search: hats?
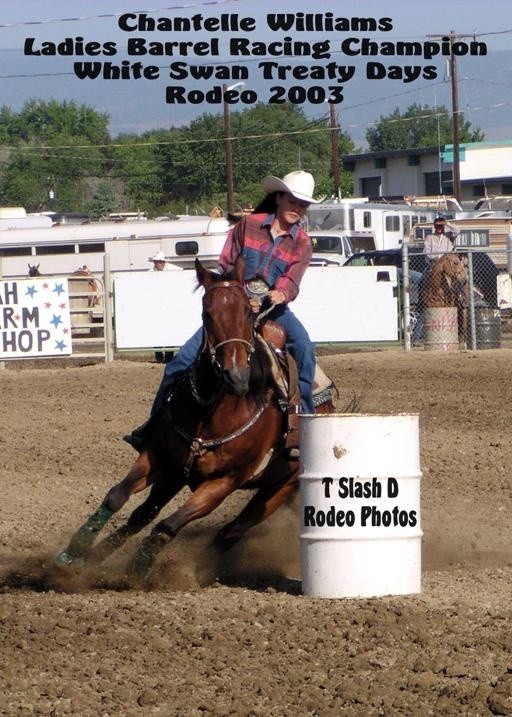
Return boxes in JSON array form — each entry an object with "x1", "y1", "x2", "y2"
[{"x1": 262, "y1": 170, "x2": 328, "y2": 204}]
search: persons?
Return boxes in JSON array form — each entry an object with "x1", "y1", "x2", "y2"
[
  {"x1": 146, "y1": 250, "x2": 175, "y2": 363},
  {"x1": 124, "y1": 169, "x2": 330, "y2": 460},
  {"x1": 457, "y1": 252, "x2": 485, "y2": 350},
  {"x1": 414, "y1": 217, "x2": 461, "y2": 313}
]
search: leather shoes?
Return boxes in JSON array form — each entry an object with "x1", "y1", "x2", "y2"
[{"x1": 122, "y1": 433, "x2": 141, "y2": 445}]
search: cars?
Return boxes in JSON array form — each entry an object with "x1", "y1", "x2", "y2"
[
  {"x1": 306, "y1": 231, "x2": 353, "y2": 266},
  {"x1": 343, "y1": 248, "x2": 499, "y2": 342}
]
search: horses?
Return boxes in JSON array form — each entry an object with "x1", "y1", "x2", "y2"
[
  {"x1": 416, "y1": 248, "x2": 469, "y2": 349},
  {"x1": 55, "y1": 251, "x2": 340, "y2": 582},
  {"x1": 70, "y1": 263, "x2": 100, "y2": 323},
  {"x1": 27, "y1": 262, "x2": 42, "y2": 277}
]
